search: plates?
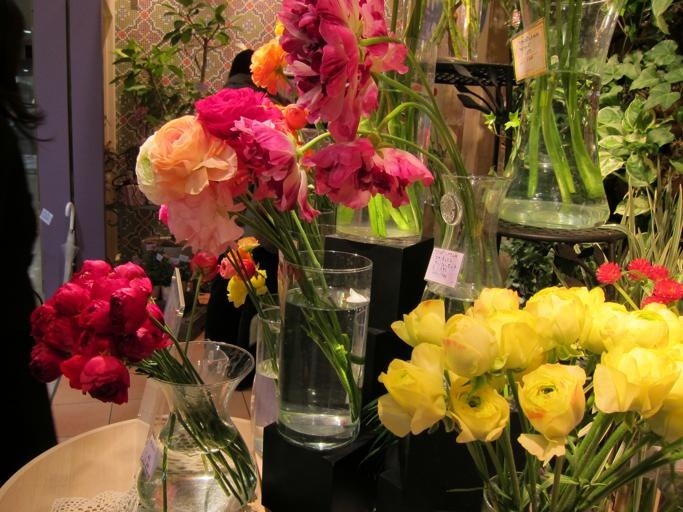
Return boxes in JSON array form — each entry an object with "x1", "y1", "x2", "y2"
[{"x1": 0, "y1": 410, "x2": 265, "y2": 512}]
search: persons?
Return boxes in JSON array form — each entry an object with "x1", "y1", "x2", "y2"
[{"x1": 2, "y1": 1, "x2": 58, "y2": 489}]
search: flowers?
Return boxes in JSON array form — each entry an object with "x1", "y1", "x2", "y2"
[{"x1": 22, "y1": 2, "x2": 680, "y2": 512}]
